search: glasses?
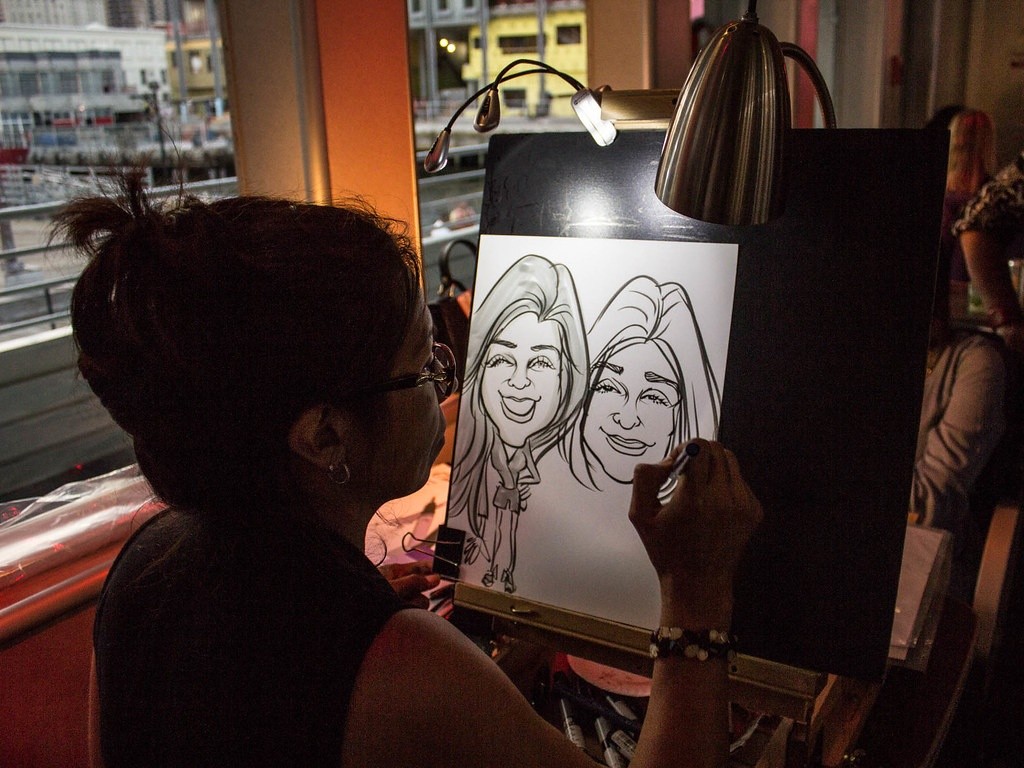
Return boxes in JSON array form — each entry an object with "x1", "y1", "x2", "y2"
[{"x1": 337, "y1": 343, "x2": 457, "y2": 406}]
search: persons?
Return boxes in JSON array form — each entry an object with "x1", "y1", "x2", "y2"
[
  {"x1": 922, "y1": 103, "x2": 1024, "y2": 352},
  {"x1": 908, "y1": 244, "x2": 1005, "y2": 523},
  {"x1": 57, "y1": 195, "x2": 762, "y2": 767}
]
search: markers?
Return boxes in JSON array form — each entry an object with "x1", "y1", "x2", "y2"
[
  {"x1": 600, "y1": 688, "x2": 643, "y2": 728},
  {"x1": 593, "y1": 715, "x2": 627, "y2": 768},
  {"x1": 606, "y1": 728, "x2": 638, "y2": 762},
  {"x1": 559, "y1": 697, "x2": 588, "y2": 753},
  {"x1": 671, "y1": 442, "x2": 700, "y2": 475}
]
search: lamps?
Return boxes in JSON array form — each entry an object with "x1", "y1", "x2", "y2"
[
  {"x1": 424, "y1": 59, "x2": 616, "y2": 172},
  {"x1": 653, "y1": 6, "x2": 833, "y2": 229}
]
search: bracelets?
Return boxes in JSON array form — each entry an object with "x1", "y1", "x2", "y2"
[
  {"x1": 649, "y1": 627, "x2": 735, "y2": 660},
  {"x1": 992, "y1": 317, "x2": 1020, "y2": 332}
]
